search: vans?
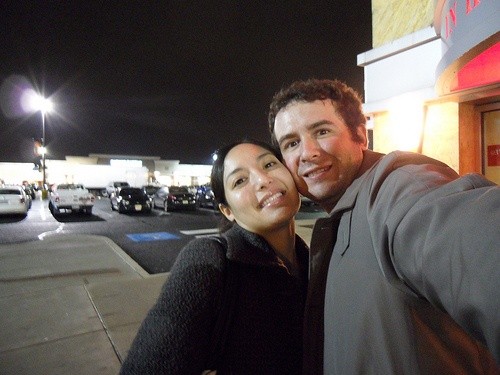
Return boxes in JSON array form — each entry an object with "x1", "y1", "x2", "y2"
[{"x1": 105, "y1": 182, "x2": 129, "y2": 197}]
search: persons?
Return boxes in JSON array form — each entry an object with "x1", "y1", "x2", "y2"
[
  {"x1": 258, "y1": 76, "x2": 500, "y2": 374},
  {"x1": 33, "y1": 138, "x2": 43, "y2": 173},
  {"x1": 116, "y1": 140, "x2": 322, "y2": 374}
]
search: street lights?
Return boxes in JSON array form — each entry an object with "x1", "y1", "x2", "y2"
[{"x1": 30, "y1": 98, "x2": 53, "y2": 191}]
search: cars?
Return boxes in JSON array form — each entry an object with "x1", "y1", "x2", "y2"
[
  {"x1": 142, "y1": 186, "x2": 161, "y2": 203},
  {"x1": 112, "y1": 187, "x2": 151, "y2": 215},
  {"x1": 189, "y1": 185, "x2": 220, "y2": 212},
  {"x1": 150, "y1": 186, "x2": 196, "y2": 214},
  {"x1": 0, "y1": 180, "x2": 35, "y2": 219}
]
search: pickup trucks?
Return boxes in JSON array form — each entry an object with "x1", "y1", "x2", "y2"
[{"x1": 48, "y1": 182, "x2": 93, "y2": 219}]
